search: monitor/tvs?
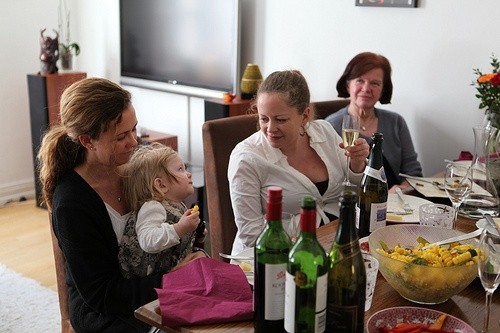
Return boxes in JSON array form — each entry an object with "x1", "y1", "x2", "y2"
[{"x1": 119, "y1": 0, "x2": 243, "y2": 98}]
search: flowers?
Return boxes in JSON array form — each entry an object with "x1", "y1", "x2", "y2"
[{"x1": 468, "y1": 52, "x2": 500, "y2": 152}]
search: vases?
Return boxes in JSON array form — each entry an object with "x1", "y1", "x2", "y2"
[{"x1": 484, "y1": 125, "x2": 500, "y2": 166}]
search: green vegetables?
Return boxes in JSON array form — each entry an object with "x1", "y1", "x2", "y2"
[{"x1": 379, "y1": 236, "x2": 477, "y2": 289}]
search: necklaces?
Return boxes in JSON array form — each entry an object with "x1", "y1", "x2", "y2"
[
  {"x1": 345, "y1": 111, "x2": 375, "y2": 131},
  {"x1": 111, "y1": 189, "x2": 123, "y2": 201}
]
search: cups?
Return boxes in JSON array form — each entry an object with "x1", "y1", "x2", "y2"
[
  {"x1": 362, "y1": 254, "x2": 379, "y2": 312},
  {"x1": 419, "y1": 204, "x2": 455, "y2": 229}
]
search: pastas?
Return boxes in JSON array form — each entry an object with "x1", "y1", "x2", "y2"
[{"x1": 374, "y1": 243, "x2": 489, "y2": 291}]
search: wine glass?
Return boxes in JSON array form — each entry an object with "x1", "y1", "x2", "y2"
[
  {"x1": 338, "y1": 115, "x2": 360, "y2": 187},
  {"x1": 478, "y1": 225, "x2": 500, "y2": 333},
  {"x1": 445, "y1": 164, "x2": 473, "y2": 230}
]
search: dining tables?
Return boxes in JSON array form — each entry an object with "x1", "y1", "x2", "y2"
[{"x1": 134, "y1": 168, "x2": 500, "y2": 333}]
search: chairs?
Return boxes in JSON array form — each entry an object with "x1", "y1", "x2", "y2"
[{"x1": 49, "y1": 99, "x2": 352, "y2": 333}]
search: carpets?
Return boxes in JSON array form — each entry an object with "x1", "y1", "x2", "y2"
[{"x1": 0, "y1": 261, "x2": 62, "y2": 333}]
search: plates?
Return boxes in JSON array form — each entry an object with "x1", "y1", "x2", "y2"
[
  {"x1": 450, "y1": 161, "x2": 487, "y2": 180},
  {"x1": 231, "y1": 248, "x2": 254, "y2": 286},
  {"x1": 476, "y1": 218, "x2": 500, "y2": 237},
  {"x1": 406, "y1": 178, "x2": 493, "y2": 197},
  {"x1": 366, "y1": 307, "x2": 476, "y2": 333},
  {"x1": 358, "y1": 235, "x2": 369, "y2": 254},
  {"x1": 386, "y1": 194, "x2": 441, "y2": 222}
]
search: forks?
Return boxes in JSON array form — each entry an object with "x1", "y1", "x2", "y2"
[{"x1": 395, "y1": 186, "x2": 414, "y2": 210}]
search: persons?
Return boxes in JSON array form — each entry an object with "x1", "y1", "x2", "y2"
[
  {"x1": 36, "y1": 77, "x2": 211, "y2": 333},
  {"x1": 116, "y1": 142, "x2": 208, "y2": 276},
  {"x1": 230, "y1": 70, "x2": 370, "y2": 294},
  {"x1": 325, "y1": 52, "x2": 423, "y2": 196}
]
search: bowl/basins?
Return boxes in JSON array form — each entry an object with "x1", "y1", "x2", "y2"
[{"x1": 369, "y1": 224, "x2": 490, "y2": 304}]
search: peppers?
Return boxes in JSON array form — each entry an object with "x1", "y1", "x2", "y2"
[{"x1": 379, "y1": 323, "x2": 455, "y2": 333}]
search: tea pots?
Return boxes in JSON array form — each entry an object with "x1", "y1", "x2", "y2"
[{"x1": 223, "y1": 93, "x2": 236, "y2": 102}]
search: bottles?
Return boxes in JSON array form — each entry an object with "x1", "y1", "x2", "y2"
[
  {"x1": 454, "y1": 125, "x2": 500, "y2": 219},
  {"x1": 253, "y1": 186, "x2": 292, "y2": 333},
  {"x1": 327, "y1": 191, "x2": 366, "y2": 333},
  {"x1": 284, "y1": 196, "x2": 328, "y2": 333},
  {"x1": 358, "y1": 133, "x2": 388, "y2": 239}
]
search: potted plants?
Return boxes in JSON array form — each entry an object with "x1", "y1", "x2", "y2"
[{"x1": 57, "y1": 42, "x2": 81, "y2": 71}]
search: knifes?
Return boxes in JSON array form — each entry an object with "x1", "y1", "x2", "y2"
[
  {"x1": 387, "y1": 211, "x2": 413, "y2": 215},
  {"x1": 484, "y1": 213, "x2": 500, "y2": 235}
]
